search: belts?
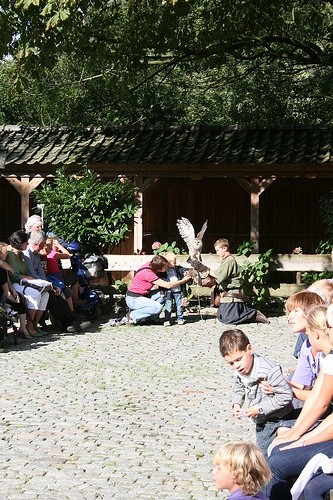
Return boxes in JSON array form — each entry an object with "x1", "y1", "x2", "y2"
[{"x1": 221, "y1": 292, "x2": 245, "y2": 300}]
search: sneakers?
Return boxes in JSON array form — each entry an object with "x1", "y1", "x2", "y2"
[{"x1": 255, "y1": 310, "x2": 269, "y2": 323}]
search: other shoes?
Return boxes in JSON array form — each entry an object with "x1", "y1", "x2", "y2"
[
  {"x1": 164, "y1": 321, "x2": 170, "y2": 326},
  {"x1": 178, "y1": 319, "x2": 184, "y2": 325},
  {"x1": 66, "y1": 321, "x2": 91, "y2": 332},
  {"x1": 27, "y1": 327, "x2": 43, "y2": 337},
  {"x1": 19, "y1": 327, "x2": 33, "y2": 339},
  {"x1": 34, "y1": 327, "x2": 48, "y2": 336},
  {"x1": 126, "y1": 312, "x2": 135, "y2": 326}
]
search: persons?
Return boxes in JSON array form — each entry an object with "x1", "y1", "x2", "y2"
[
  {"x1": 212, "y1": 441, "x2": 272, "y2": 500},
  {"x1": 156, "y1": 253, "x2": 190, "y2": 327},
  {"x1": 126, "y1": 255, "x2": 192, "y2": 328},
  {"x1": 265, "y1": 278, "x2": 333, "y2": 500},
  {"x1": 22, "y1": 234, "x2": 91, "y2": 332},
  {"x1": 219, "y1": 329, "x2": 297, "y2": 461},
  {"x1": 4, "y1": 230, "x2": 49, "y2": 337},
  {"x1": 0, "y1": 242, "x2": 32, "y2": 338},
  {"x1": 194, "y1": 238, "x2": 270, "y2": 326},
  {"x1": 24, "y1": 215, "x2": 87, "y2": 322}
]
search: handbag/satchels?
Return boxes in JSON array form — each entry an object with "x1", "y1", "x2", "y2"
[{"x1": 20, "y1": 278, "x2": 53, "y2": 293}]
